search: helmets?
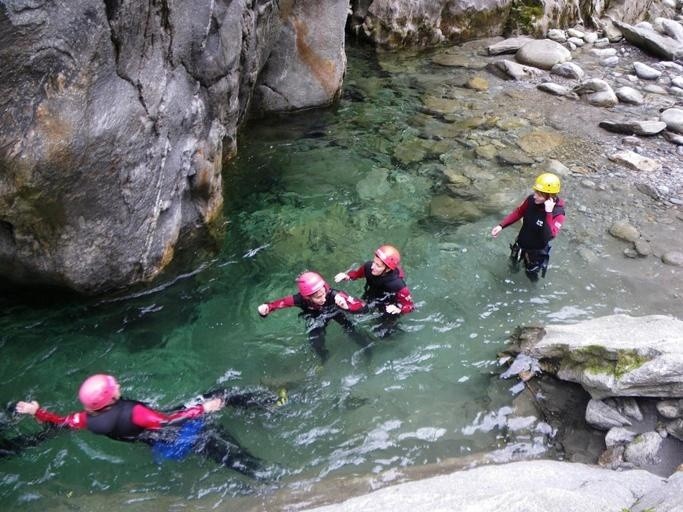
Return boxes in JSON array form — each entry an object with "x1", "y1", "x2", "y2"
[
  {"x1": 532, "y1": 173, "x2": 560, "y2": 193},
  {"x1": 77, "y1": 373, "x2": 117, "y2": 411},
  {"x1": 375, "y1": 245, "x2": 400, "y2": 271},
  {"x1": 298, "y1": 272, "x2": 325, "y2": 297}
]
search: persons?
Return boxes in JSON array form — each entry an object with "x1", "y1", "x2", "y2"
[
  {"x1": 334, "y1": 245, "x2": 417, "y2": 341},
  {"x1": 491, "y1": 174, "x2": 565, "y2": 286},
  {"x1": 259, "y1": 272, "x2": 377, "y2": 367},
  {"x1": 17, "y1": 373, "x2": 292, "y2": 485}
]
